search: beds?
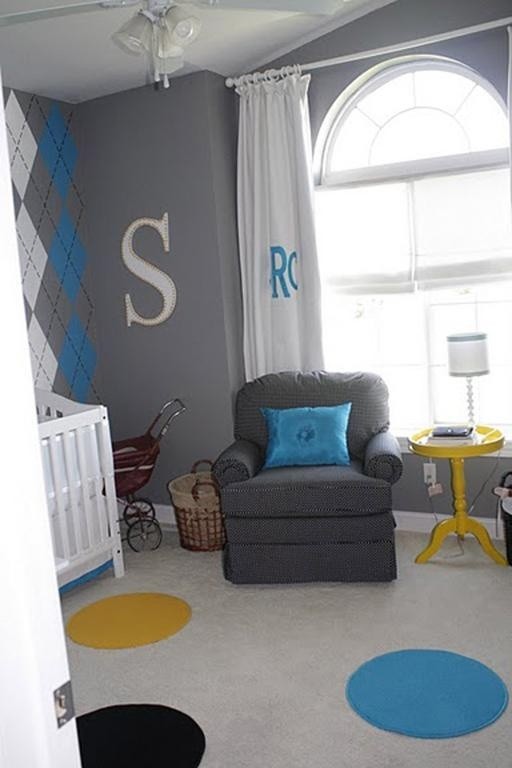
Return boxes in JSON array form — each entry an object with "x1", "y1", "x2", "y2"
[{"x1": 34, "y1": 388, "x2": 125, "y2": 595}]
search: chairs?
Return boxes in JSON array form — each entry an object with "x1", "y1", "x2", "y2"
[{"x1": 213, "y1": 370, "x2": 403, "y2": 585}]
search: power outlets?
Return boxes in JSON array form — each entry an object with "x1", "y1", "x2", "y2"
[{"x1": 424, "y1": 463, "x2": 436, "y2": 483}]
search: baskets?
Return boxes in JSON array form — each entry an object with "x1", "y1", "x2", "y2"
[{"x1": 168, "y1": 471, "x2": 225, "y2": 551}]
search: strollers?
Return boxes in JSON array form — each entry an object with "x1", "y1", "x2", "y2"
[{"x1": 101, "y1": 397, "x2": 187, "y2": 552}]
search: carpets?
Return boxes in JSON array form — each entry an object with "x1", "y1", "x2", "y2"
[
  {"x1": 76, "y1": 704, "x2": 205, "y2": 768},
  {"x1": 346, "y1": 650, "x2": 508, "y2": 740},
  {"x1": 66, "y1": 593, "x2": 192, "y2": 650}
]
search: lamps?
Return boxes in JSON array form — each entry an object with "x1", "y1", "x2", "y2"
[
  {"x1": 450, "y1": 334, "x2": 490, "y2": 427},
  {"x1": 114, "y1": 5, "x2": 203, "y2": 88}
]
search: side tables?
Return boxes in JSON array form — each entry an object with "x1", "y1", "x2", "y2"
[{"x1": 408, "y1": 425, "x2": 506, "y2": 563}]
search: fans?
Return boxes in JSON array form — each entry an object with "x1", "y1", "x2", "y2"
[{"x1": 0, "y1": 1, "x2": 345, "y2": 26}]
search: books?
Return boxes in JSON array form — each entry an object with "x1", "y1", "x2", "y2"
[
  {"x1": 432, "y1": 424, "x2": 473, "y2": 437},
  {"x1": 428, "y1": 426, "x2": 477, "y2": 440}
]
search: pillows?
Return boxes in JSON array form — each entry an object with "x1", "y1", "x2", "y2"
[{"x1": 259, "y1": 402, "x2": 354, "y2": 471}]
